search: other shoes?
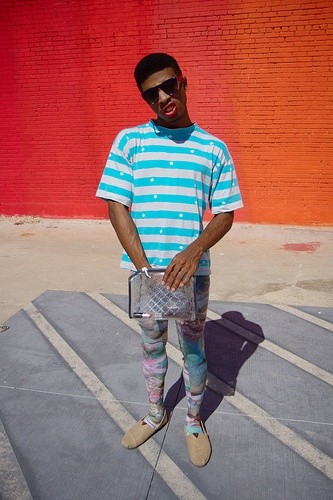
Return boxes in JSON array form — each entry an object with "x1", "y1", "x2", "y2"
[
  {"x1": 121, "y1": 409, "x2": 168, "y2": 449},
  {"x1": 184, "y1": 418, "x2": 212, "y2": 467}
]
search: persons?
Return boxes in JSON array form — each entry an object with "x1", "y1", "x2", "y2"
[{"x1": 94, "y1": 51, "x2": 244, "y2": 469}]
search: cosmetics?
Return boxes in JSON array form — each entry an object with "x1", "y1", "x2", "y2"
[{"x1": 161, "y1": 310, "x2": 189, "y2": 319}]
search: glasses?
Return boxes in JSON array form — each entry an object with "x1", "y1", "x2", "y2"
[{"x1": 141, "y1": 72, "x2": 182, "y2": 105}]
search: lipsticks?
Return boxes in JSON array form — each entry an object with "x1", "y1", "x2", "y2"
[{"x1": 132, "y1": 312, "x2": 150, "y2": 318}]
